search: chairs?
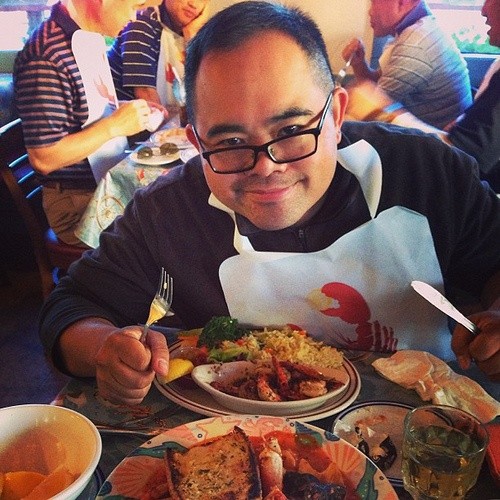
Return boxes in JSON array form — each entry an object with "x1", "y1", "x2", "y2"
[{"x1": 0, "y1": 117, "x2": 94, "y2": 303}]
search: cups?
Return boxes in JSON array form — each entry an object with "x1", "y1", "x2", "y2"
[{"x1": 399, "y1": 404, "x2": 490, "y2": 500}]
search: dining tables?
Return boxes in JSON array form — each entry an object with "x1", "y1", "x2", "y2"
[
  {"x1": 110, "y1": 110, "x2": 201, "y2": 193},
  {"x1": 0, "y1": 309, "x2": 500, "y2": 500}
]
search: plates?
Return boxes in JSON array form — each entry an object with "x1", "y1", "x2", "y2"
[
  {"x1": 130, "y1": 145, "x2": 181, "y2": 165},
  {"x1": 154, "y1": 330, "x2": 362, "y2": 421},
  {"x1": 327, "y1": 400, "x2": 469, "y2": 490},
  {"x1": 93, "y1": 415, "x2": 399, "y2": 500},
  {"x1": 181, "y1": 149, "x2": 201, "y2": 165},
  {"x1": 150, "y1": 128, "x2": 196, "y2": 149},
  {"x1": 192, "y1": 363, "x2": 350, "y2": 415}
]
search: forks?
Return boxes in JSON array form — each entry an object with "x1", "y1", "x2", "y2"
[
  {"x1": 139, "y1": 267, "x2": 175, "y2": 342},
  {"x1": 334, "y1": 39, "x2": 362, "y2": 88}
]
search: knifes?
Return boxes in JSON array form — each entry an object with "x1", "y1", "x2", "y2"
[{"x1": 411, "y1": 280, "x2": 482, "y2": 337}]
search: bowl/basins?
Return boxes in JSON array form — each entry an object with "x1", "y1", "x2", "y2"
[{"x1": 0, "y1": 399, "x2": 102, "y2": 500}]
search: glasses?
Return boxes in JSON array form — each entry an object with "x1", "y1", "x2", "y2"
[{"x1": 191, "y1": 92, "x2": 333, "y2": 175}]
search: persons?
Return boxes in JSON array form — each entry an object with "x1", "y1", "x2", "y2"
[
  {"x1": 341, "y1": 0, "x2": 474, "y2": 130},
  {"x1": 39, "y1": 0, "x2": 500, "y2": 405},
  {"x1": 108, "y1": 0, "x2": 206, "y2": 147},
  {"x1": 12, "y1": 0, "x2": 171, "y2": 246},
  {"x1": 342, "y1": 1, "x2": 500, "y2": 196}
]
